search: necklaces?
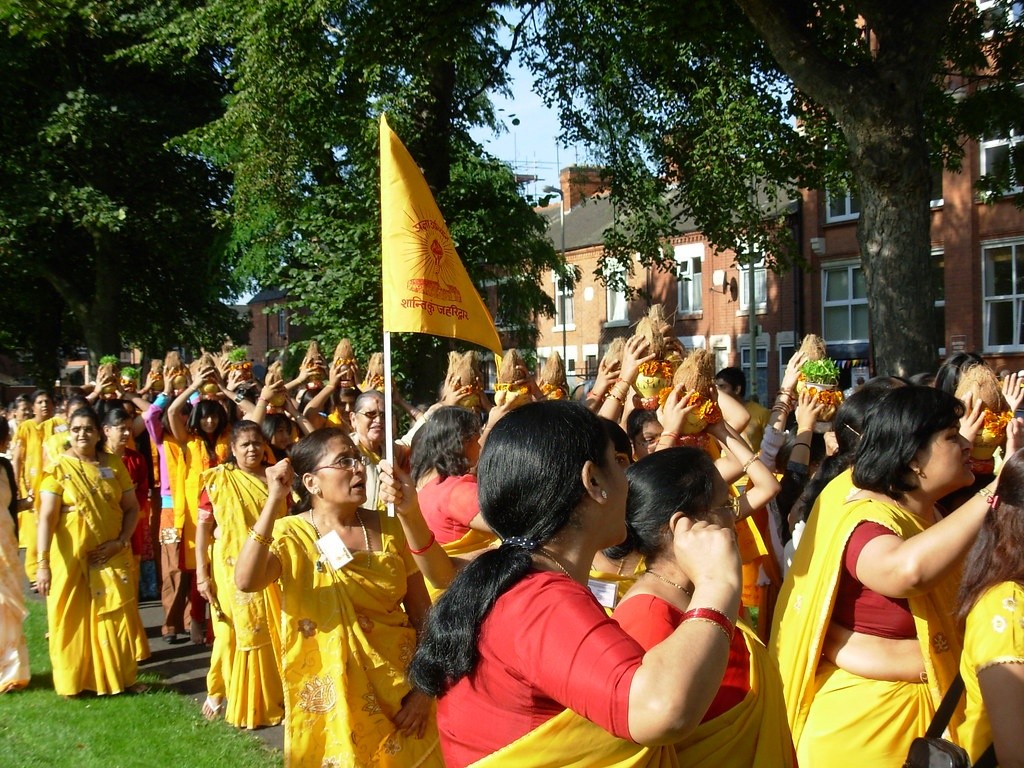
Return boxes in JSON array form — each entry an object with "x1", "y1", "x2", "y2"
[
  {"x1": 646, "y1": 570, "x2": 692, "y2": 598},
  {"x1": 79, "y1": 455, "x2": 101, "y2": 494},
  {"x1": 309, "y1": 508, "x2": 370, "y2": 568},
  {"x1": 538, "y1": 549, "x2": 573, "y2": 580}
]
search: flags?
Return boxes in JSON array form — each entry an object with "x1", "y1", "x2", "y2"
[{"x1": 380, "y1": 115, "x2": 504, "y2": 356}]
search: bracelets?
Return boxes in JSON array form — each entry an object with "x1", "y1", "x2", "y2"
[
  {"x1": 772, "y1": 406, "x2": 788, "y2": 415},
  {"x1": 775, "y1": 401, "x2": 790, "y2": 411},
  {"x1": 979, "y1": 488, "x2": 994, "y2": 499},
  {"x1": 196, "y1": 577, "x2": 210, "y2": 585},
  {"x1": 743, "y1": 456, "x2": 758, "y2": 472},
  {"x1": 777, "y1": 388, "x2": 793, "y2": 398},
  {"x1": 189, "y1": 385, "x2": 198, "y2": 391},
  {"x1": 295, "y1": 413, "x2": 303, "y2": 420},
  {"x1": 661, "y1": 431, "x2": 680, "y2": 440},
  {"x1": 406, "y1": 530, "x2": 435, "y2": 554},
  {"x1": 37, "y1": 552, "x2": 50, "y2": 568},
  {"x1": 793, "y1": 443, "x2": 811, "y2": 450},
  {"x1": 411, "y1": 408, "x2": 420, "y2": 417},
  {"x1": 234, "y1": 395, "x2": 245, "y2": 404},
  {"x1": 604, "y1": 393, "x2": 624, "y2": 406},
  {"x1": 614, "y1": 386, "x2": 626, "y2": 400},
  {"x1": 679, "y1": 608, "x2": 735, "y2": 642},
  {"x1": 257, "y1": 398, "x2": 269, "y2": 403},
  {"x1": 248, "y1": 528, "x2": 274, "y2": 546}
]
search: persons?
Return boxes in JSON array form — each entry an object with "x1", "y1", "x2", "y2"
[{"x1": 0, "y1": 314, "x2": 1024, "y2": 768}]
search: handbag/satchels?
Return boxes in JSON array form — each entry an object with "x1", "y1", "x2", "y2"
[{"x1": 903, "y1": 738, "x2": 972, "y2": 768}]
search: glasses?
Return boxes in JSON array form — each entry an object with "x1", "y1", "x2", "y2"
[
  {"x1": 69, "y1": 426, "x2": 98, "y2": 433},
  {"x1": 354, "y1": 409, "x2": 384, "y2": 418},
  {"x1": 309, "y1": 455, "x2": 371, "y2": 473},
  {"x1": 698, "y1": 498, "x2": 742, "y2": 518},
  {"x1": 634, "y1": 438, "x2": 662, "y2": 450}
]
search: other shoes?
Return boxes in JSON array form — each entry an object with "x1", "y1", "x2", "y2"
[
  {"x1": 30, "y1": 579, "x2": 40, "y2": 592},
  {"x1": 6, "y1": 685, "x2": 19, "y2": 694},
  {"x1": 163, "y1": 634, "x2": 176, "y2": 644}
]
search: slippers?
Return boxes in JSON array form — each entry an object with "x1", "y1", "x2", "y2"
[{"x1": 202, "y1": 693, "x2": 223, "y2": 724}]
